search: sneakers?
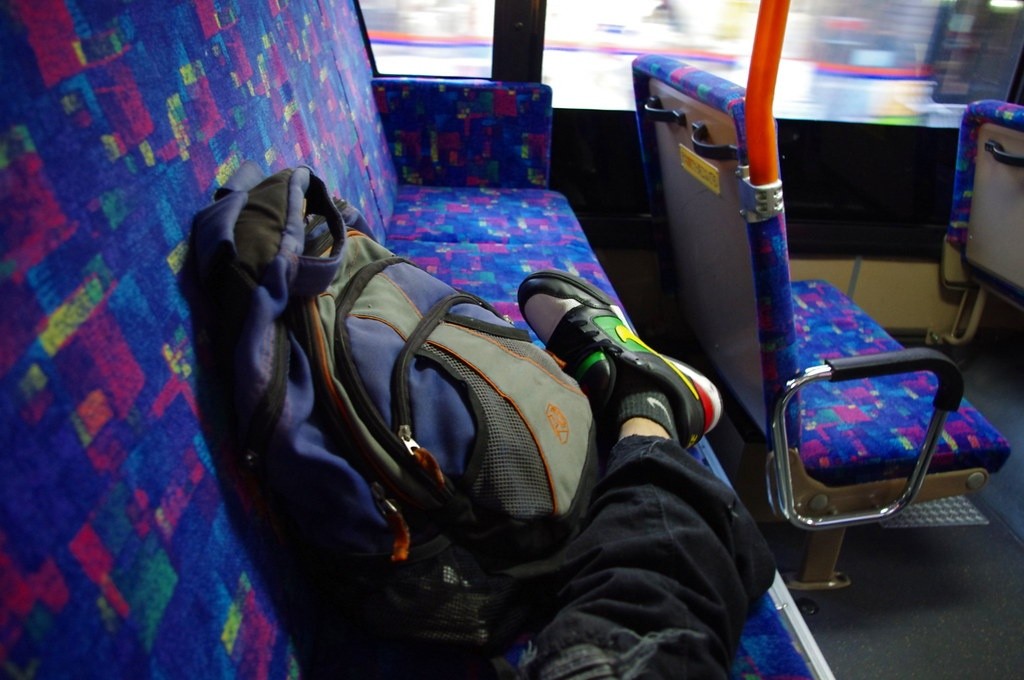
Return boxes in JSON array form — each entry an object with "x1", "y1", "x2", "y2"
[{"x1": 518, "y1": 267, "x2": 724, "y2": 451}]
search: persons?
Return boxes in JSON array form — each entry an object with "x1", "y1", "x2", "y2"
[{"x1": 503, "y1": 269, "x2": 779, "y2": 679}]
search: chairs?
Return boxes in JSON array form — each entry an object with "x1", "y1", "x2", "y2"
[
  {"x1": 626, "y1": 53, "x2": 1016, "y2": 597},
  {"x1": 926, "y1": 90, "x2": 1024, "y2": 340}
]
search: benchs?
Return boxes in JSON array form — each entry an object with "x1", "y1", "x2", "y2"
[{"x1": 0, "y1": 0, "x2": 838, "y2": 680}]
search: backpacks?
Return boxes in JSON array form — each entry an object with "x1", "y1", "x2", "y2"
[{"x1": 182, "y1": 165, "x2": 605, "y2": 653}]
